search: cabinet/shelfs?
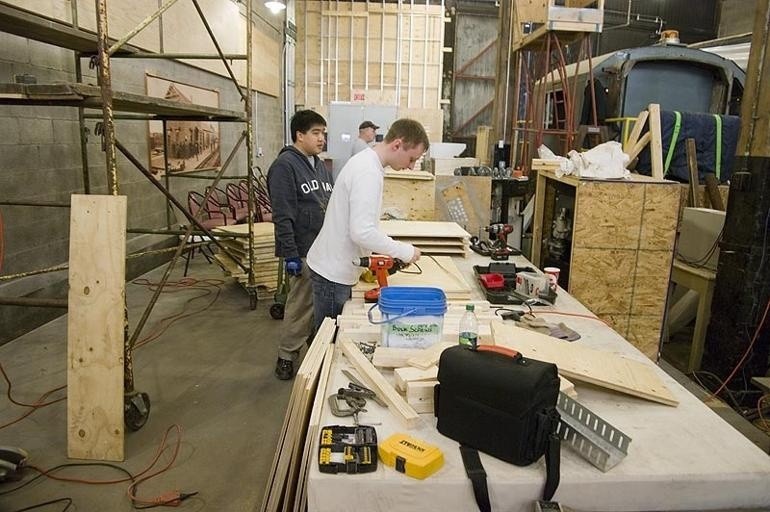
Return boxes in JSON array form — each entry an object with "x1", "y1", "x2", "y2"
[{"x1": 531, "y1": 170, "x2": 682, "y2": 363}]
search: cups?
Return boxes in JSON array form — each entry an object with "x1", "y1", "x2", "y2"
[{"x1": 544, "y1": 267, "x2": 560, "y2": 293}]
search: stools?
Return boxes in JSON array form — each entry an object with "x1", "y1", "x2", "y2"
[{"x1": 672, "y1": 259, "x2": 715, "y2": 379}]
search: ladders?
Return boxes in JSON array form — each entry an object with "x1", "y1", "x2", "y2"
[{"x1": 550, "y1": 30, "x2": 568, "y2": 157}]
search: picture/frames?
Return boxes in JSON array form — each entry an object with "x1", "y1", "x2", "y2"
[{"x1": 145, "y1": 72, "x2": 222, "y2": 178}]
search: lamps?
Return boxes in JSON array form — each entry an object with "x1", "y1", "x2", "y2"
[{"x1": 265, "y1": 1, "x2": 286, "y2": 10}]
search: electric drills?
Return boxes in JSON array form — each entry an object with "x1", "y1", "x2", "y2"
[
  {"x1": 352, "y1": 255, "x2": 395, "y2": 304},
  {"x1": 481, "y1": 224, "x2": 514, "y2": 254}
]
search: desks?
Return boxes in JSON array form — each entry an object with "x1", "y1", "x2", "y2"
[{"x1": 305, "y1": 247, "x2": 769, "y2": 512}]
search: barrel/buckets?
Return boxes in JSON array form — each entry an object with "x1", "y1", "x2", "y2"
[{"x1": 366, "y1": 285, "x2": 449, "y2": 350}]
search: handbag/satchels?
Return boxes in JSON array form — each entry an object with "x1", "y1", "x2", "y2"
[
  {"x1": 636, "y1": 108, "x2": 740, "y2": 184},
  {"x1": 433, "y1": 344, "x2": 561, "y2": 467}
]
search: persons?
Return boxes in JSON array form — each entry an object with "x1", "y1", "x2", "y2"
[
  {"x1": 351, "y1": 120, "x2": 380, "y2": 154},
  {"x1": 306, "y1": 118, "x2": 431, "y2": 331},
  {"x1": 266, "y1": 108, "x2": 335, "y2": 382}
]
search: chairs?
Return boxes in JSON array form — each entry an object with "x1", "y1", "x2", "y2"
[{"x1": 187, "y1": 175, "x2": 273, "y2": 264}]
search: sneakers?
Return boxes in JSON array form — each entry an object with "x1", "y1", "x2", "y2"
[{"x1": 276, "y1": 357, "x2": 293, "y2": 380}]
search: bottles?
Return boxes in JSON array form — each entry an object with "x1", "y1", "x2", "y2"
[{"x1": 459, "y1": 303, "x2": 479, "y2": 350}]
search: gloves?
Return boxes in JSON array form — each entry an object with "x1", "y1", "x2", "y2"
[{"x1": 285, "y1": 257, "x2": 304, "y2": 276}]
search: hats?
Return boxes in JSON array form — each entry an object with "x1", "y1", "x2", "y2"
[{"x1": 359, "y1": 120, "x2": 380, "y2": 129}]
